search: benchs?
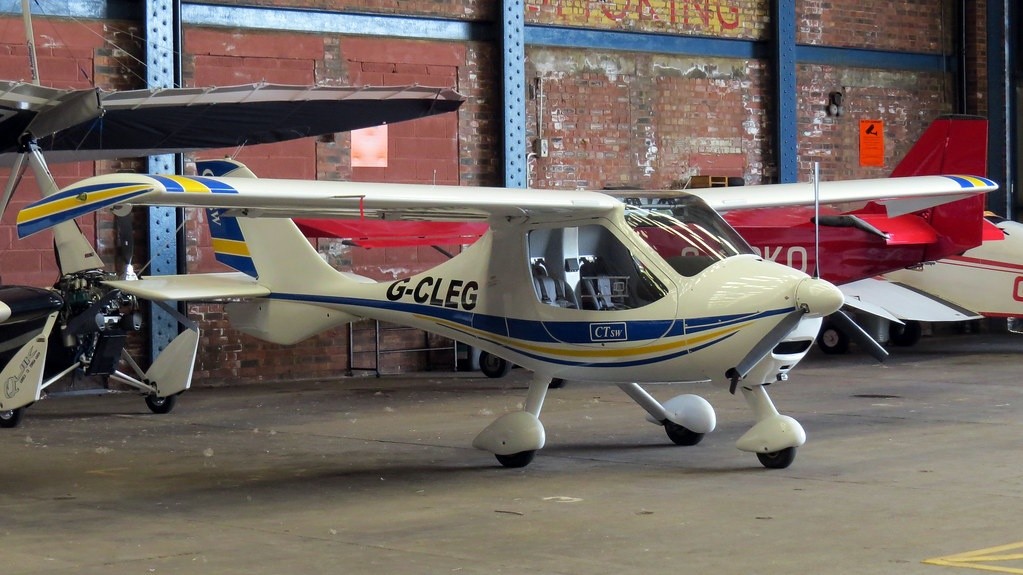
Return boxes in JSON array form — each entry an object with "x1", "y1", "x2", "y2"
[{"x1": 531, "y1": 260, "x2": 580, "y2": 309}]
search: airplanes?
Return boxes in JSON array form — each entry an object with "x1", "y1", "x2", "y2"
[{"x1": 0, "y1": 0, "x2": 1023, "y2": 472}]
